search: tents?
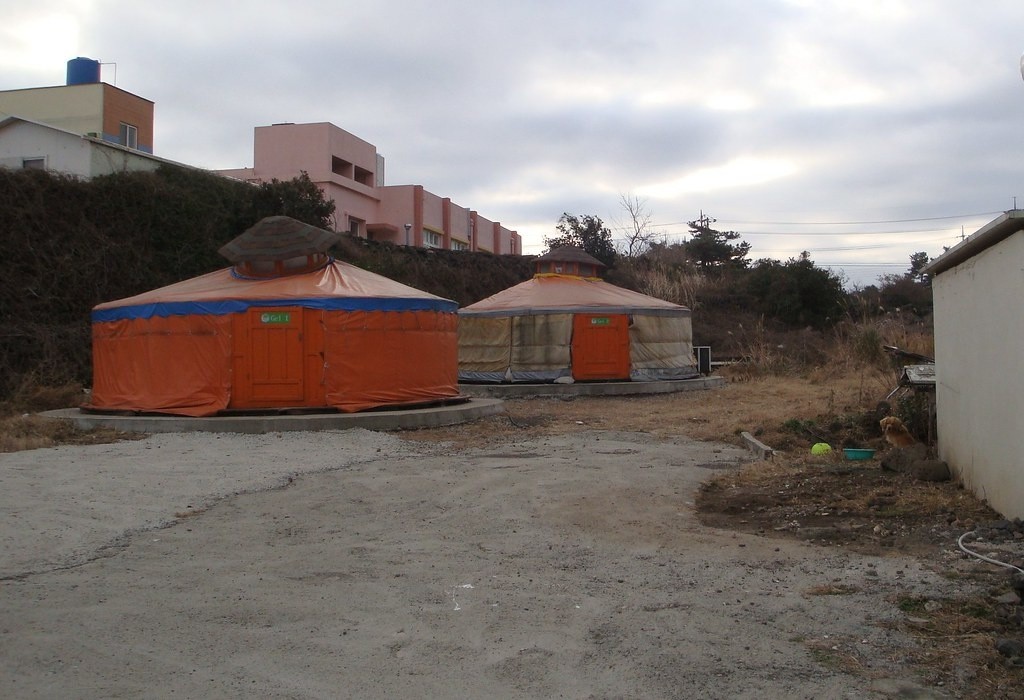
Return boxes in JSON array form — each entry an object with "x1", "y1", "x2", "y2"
[
  {"x1": 90, "y1": 216, "x2": 462, "y2": 417},
  {"x1": 461, "y1": 245, "x2": 697, "y2": 382}
]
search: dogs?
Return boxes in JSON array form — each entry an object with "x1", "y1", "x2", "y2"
[{"x1": 880, "y1": 416, "x2": 917, "y2": 452}]
